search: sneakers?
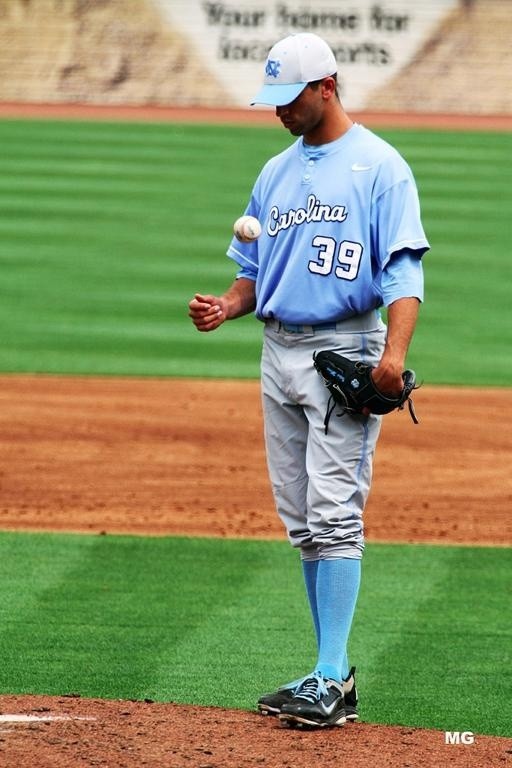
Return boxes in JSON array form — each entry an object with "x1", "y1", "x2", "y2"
[
  {"x1": 258, "y1": 667, "x2": 358, "y2": 721},
  {"x1": 281, "y1": 676, "x2": 345, "y2": 726}
]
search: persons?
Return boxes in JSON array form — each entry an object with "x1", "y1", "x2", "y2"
[{"x1": 188, "y1": 31, "x2": 431, "y2": 730}]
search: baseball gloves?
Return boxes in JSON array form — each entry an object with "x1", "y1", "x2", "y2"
[{"x1": 312, "y1": 350, "x2": 416, "y2": 415}]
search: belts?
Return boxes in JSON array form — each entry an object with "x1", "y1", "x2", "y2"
[{"x1": 278, "y1": 320, "x2": 336, "y2": 333}]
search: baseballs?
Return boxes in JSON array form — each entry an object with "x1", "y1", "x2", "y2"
[{"x1": 233, "y1": 216, "x2": 262, "y2": 243}]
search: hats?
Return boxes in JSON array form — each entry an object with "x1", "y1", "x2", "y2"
[{"x1": 250, "y1": 32, "x2": 336, "y2": 105}]
